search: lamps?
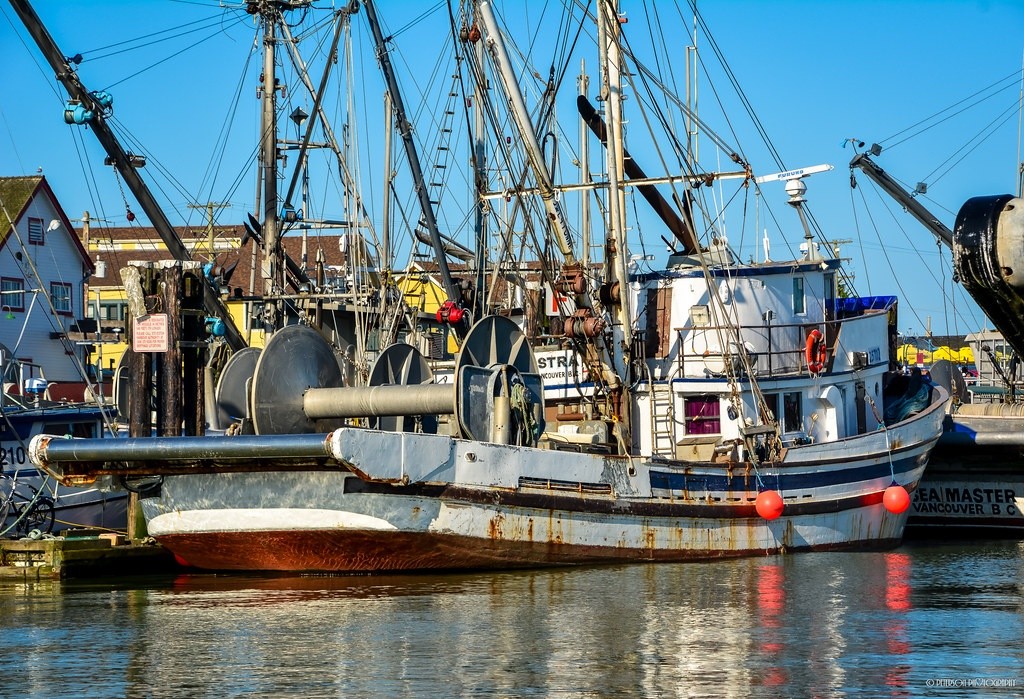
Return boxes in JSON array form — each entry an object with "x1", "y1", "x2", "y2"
[{"x1": 689, "y1": 305, "x2": 712, "y2": 352}]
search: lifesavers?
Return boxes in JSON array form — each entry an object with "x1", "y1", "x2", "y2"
[{"x1": 807, "y1": 330, "x2": 826, "y2": 372}]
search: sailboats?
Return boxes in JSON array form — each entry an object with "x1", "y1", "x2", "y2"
[{"x1": 1, "y1": 0, "x2": 1024, "y2": 583}]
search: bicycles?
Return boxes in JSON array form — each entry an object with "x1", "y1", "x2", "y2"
[{"x1": 0, "y1": 474, "x2": 57, "y2": 538}]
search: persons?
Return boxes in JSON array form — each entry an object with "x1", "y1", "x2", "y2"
[{"x1": 738, "y1": 417, "x2": 757, "y2": 462}]
